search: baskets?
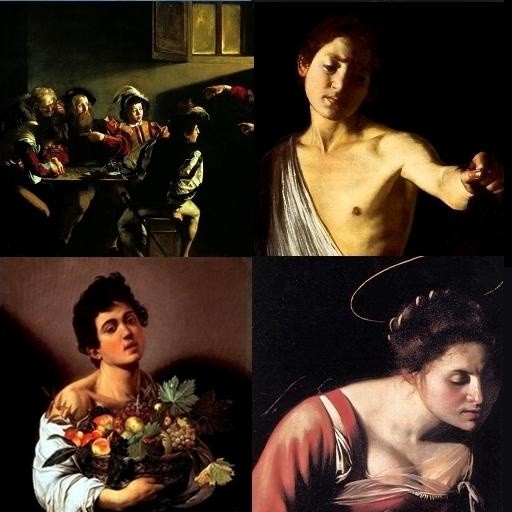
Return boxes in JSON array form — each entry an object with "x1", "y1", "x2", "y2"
[{"x1": 83, "y1": 453, "x2": 192, "y2": 508}]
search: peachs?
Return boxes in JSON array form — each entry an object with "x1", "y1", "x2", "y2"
[
  {"x1": 91, "y1": 437, "x2": 110, "y2": 456},
  {"x1": 93, "y1": 414, "x2": 114, "y2": 430}
]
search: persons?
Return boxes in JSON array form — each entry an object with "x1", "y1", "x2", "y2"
[
  {"x1": 30, "y1": 269, "x2": 217, "y2": 512},
  {"x1": 249, "y1": 289, "x2": 505, "y2": 511},
  {"x1": 267, "y1": 17, "x2": 508, "y2": 256}
]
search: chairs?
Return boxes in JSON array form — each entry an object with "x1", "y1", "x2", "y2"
[{"x1": 119, "y1": 191, "x2": 178, "y2": 256}]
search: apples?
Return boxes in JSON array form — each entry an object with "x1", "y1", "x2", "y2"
[{"x1": 125, "y1": 416, "x2": 145, "y2": 435}]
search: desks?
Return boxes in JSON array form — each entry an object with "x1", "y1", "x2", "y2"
[{"x1": 41, "y1": 164, "x2": 129, "y2": 246}]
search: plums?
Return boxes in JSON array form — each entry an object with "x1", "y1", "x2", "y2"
[{"x1": 65, "y1": 427, "x2": 105, "y2": 447}]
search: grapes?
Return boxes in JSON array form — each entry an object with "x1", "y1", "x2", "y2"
[
  {"x1": 166, "y1": 426, "x2": 197, "y2": 450},
  {"x1": 119, "y1": 400, "x2": 152, "y2": 424}
]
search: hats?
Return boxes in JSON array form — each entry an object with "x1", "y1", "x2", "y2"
[
  {"x1": 168, "y1": 113, "x2": 199, "y2": 141},
  {"x1": 119, "y1": 95, "x2": 150, "y2": 120},
  {"x1": 63, "y1": 87, "x2": 96, "y2": 107}
]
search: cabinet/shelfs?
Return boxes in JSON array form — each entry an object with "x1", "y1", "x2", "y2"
[{"x1": 146, "y1": 1, "x2": 255, "y2": 63}]
